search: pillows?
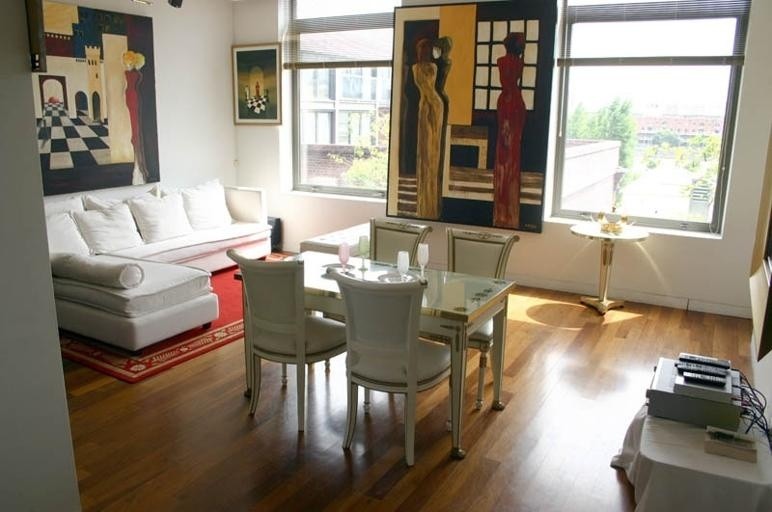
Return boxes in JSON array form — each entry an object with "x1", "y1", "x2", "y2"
[
  {"x1": 72, "y1": 203, "x2": 144, "y2": 257},
  {"x1": 181, "y1": 180, "x2": 234, "y2": 230},
  {"x1": 44, "y1": 213, "x2": 92, "y2": 257},
  {"x1": 128, "y1": 193, "x2": 193, "y2": 243}
]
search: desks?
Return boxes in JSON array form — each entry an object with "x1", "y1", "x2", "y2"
[
  {"x1": 570, "y1": 221, "x2": 651, "y2": 315},
  {"x1": 610, "y1": 385, "x2": 772, "y2": 511}
]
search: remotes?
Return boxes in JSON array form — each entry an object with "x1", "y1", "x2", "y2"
[
  {"x1": 679, "y1": 352, "x2": 731, "y2": 370},
  {"x1": 683, "y1": 371, "x2": 726, "y2": 389},
  {"x1": 674, "y1": 360, "x2": 727, "y2": 378}
]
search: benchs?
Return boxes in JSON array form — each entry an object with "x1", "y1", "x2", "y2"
[{"x1": 300, "y1": 222, "x2": 373, "y2": 258}]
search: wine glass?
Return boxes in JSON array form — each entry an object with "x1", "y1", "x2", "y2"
[
  {"x1": 338, "y1": 240, "x2": 349, "y2": 273},
  {"x1": 418, "y1": 244, "x2": 429, "y2": 280},
  {"x1": 359, "y1": 236, "x2": 369, "y2": 270},
  {"x1": 398, "y1": 251, "x2": 409, "y2": 275}
]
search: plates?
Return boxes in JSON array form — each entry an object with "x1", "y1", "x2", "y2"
[{"x1": 377, "y1": 274, "x2": 415, "y2": 283}]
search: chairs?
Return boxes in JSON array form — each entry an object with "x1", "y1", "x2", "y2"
[
  {"x1": 235, "y1": 251, "x2": 518, "y2": 459},
  {"x1": 225, "y1": 248, "x2": 346, "y2": 436},
  {"x1": 444, "y1": 228, "x2": 520, "y2": 411},
  {"x1": 366, "y1": 218, "x2": 435, "y2": 264},
  {"x1": 331, "y1": 272, "x2": 452, "y2": 465}
]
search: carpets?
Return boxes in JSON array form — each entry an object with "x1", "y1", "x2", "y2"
[{"x1": 61, "y1": 252, "x2": 311, "y2": 385}]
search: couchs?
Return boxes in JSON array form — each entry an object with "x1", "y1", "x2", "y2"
[{"x1": 44, "y1": 183, "x2": 274, "y2": 359}]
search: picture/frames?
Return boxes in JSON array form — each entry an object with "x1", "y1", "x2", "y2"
[
  {"x1": 231, "y1": 41, "x2": 284, "y2": 125},
  {"x1": 385, "y1": 0, "x2": 559, "y2": 234}
]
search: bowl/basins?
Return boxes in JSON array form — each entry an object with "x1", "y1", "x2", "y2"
[{"x1": 606, "y1": 213, "x2": 620, "y2": 224}]
